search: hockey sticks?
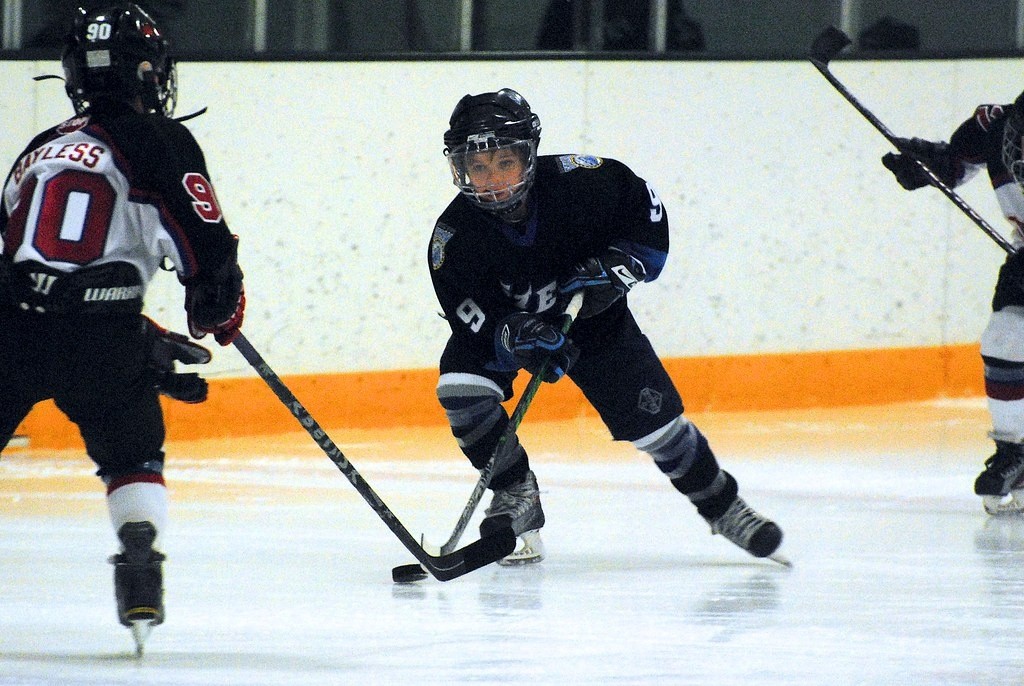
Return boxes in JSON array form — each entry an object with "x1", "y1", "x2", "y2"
[
  {"x1": 420, "y1": 291, "x2": 585, "y2": 579},
  {"x1": 230, "y1": 330, "x2": 518, "y2": 585},
  {"x1": 804, "y1": 22, "x2": 1018, "y2": 255}
]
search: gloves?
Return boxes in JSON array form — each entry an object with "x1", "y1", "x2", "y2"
[
  {"x1": 188, "y1": 291, "x2": 246, "y2": 346},
  {"x1": 883, "y1": 137, "x2": 954, "y2": 191},
  {"x1": 494, "y1": 312, "x2": 579, "y2": 384},
  {"x1": 142, "y1": 318, "x2": 211, "y2": 404},
  {"x1": 556, "y1": 251, "x2": 647, "y2": 318}
]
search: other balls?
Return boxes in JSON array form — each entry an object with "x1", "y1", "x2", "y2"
[{"x1": 391, "y1": 563, "x2": 430, "y2": 584}]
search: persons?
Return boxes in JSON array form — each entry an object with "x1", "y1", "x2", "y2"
[
  {"x1": 427, "y1": 88, "x2": 791, "y2": 568},
  {"x1": 0, "y1": 0, "x2": 246, "y2": 656},
  {"x1": 880, "y1": 92, "x2": 1024, "y2": 518}
]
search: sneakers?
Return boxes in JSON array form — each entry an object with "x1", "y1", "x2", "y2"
[
  {"x1": 480, "y1": 471, "x2": 545, "y2": 564},
  {"x1": 105, "y1": 522, "x2": 168, "y2": 656},
  {"x1": 709, "y1": 495, "x2": 791, "y2": 567},
  {"x1": 973, "y1": 431, "x2": 1024, "y2": 516}
]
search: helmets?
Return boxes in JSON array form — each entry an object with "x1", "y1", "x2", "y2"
[
  {"x1": 59, "y1": 1, "x2": 178, "y2": 117},
  {"x1": 444, "y1": 89, "x2": 542, "y2": 222}
]
